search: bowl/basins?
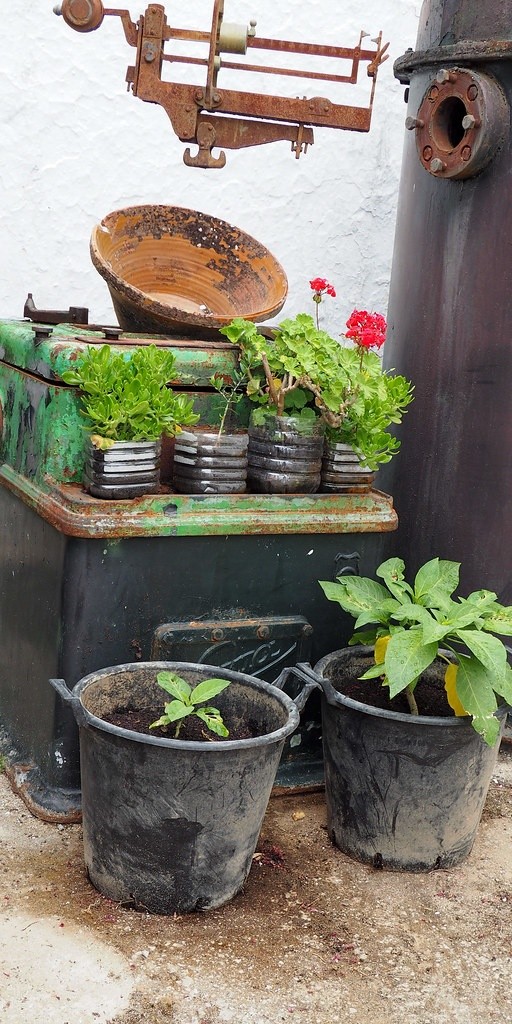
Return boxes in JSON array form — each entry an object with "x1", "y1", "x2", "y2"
[{"x1": 91, "y1": 206, "x2": 290, "y2": 339}]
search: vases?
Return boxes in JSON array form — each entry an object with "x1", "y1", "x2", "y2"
[{"x1": 321, "y1": 441, "x2": 375, "y2": 493}]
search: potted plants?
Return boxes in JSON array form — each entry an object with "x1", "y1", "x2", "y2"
[
  {"x1": 62, "y1": 343, "x2": 201, "y2": 500},
  {"x1": 218, "y1": 311, "x2": 358, "y2": 494},
  {"x1": 49, "y1": 662, "x2": 325, "y2": 916},
  {"x1": 170, "y1": 353, "x2": 262, "y2": 495},
  {"x1": 297, "y1": 558, "x2": 512, "y2": 873}
]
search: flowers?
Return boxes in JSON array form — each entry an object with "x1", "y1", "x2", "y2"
[{"x1": 307, "y1": 277, "x2": 416, "y2": 471}]
